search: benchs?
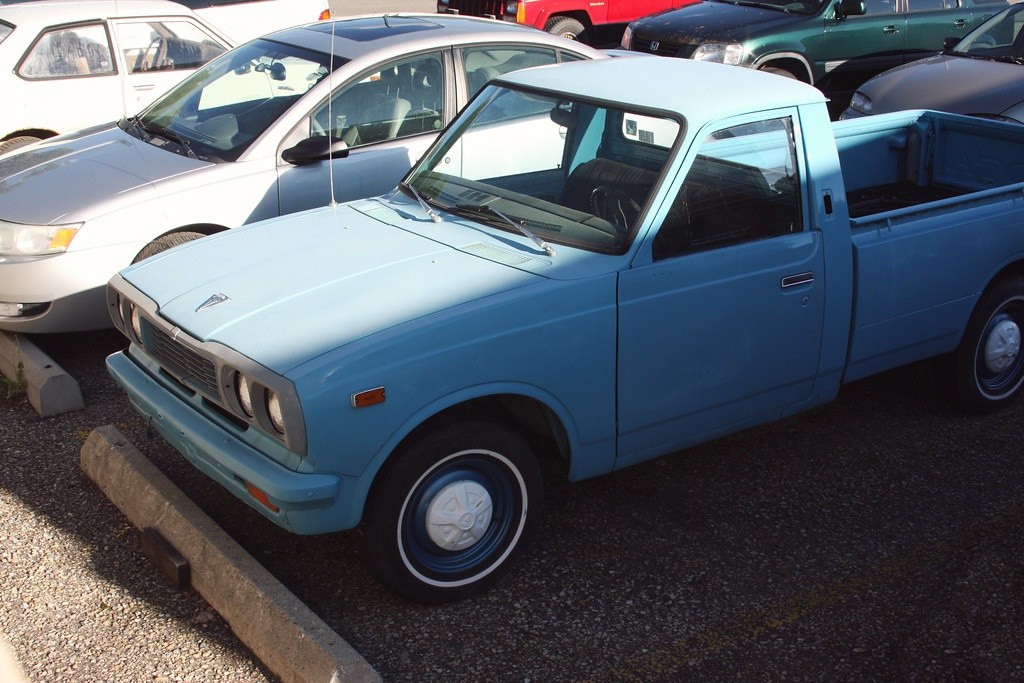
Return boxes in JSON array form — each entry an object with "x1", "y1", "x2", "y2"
[{"x1": 573, "y1": 159, "x2": 752, "y2": 251}]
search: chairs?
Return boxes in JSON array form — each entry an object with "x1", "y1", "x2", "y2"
[{"x1": 342, "y1": 63, "x2": 414, "y2": 146}]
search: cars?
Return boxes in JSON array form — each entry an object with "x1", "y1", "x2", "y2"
[
  {"x1": 621, "y1": 0, "x2": 1022, "y2": 114},
  {"x1": 433, "y1": 0, "x2": 707, "y2": 46},
  {"x1": 836, "y1": 2, "x2": 1024, "y2": 124},
  {"x1": 0, "y1": 11, "x2": 733, "y2": 338}
]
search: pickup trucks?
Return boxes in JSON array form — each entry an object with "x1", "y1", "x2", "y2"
[
  {"x1": 102, "y1": 49, "x2": 1024, "y2": 598},
  {"x1": 1, "y1": 0, "x2": 419, "y2": 163}
]
